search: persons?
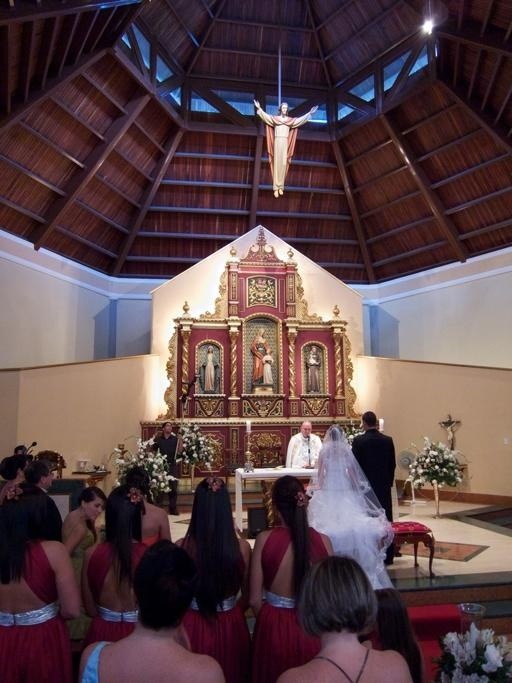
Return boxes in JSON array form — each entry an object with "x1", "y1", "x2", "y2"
[
  {"x1": 286, "y1": 421, "x2": 324, "y2": 469},
  {"x1": 1, "y1": 456, "x2": 251, "y2": 683},
  {"x1": 276, "y1": 555, "x2": 414, "y2": 683},
  {"x1": 254, "y1": 100, "x2": 318, "y2": 199},
  {"x1": 306, "y1": 345, "x2": 321, "y2": 393},
  {"x1": 150, "y1": 422, "x2": 181, "y2": 515},
  {"x1": 262, "y1": 348, "x2": 273, "y2": 384},
  {"x1": 361, "y1": 588, "x2": 425, "y2": 683},
  {"x1": 250, "y1": 328, "x2": 269, "y2": 385},
  {"x1": 203, "y1": 346, "x2": 218, "y2": 392},
  {"x1": 248, "y1": 475, "x2": 335, "y2": 683},
  {"x1": 351, "y1": 411, "x2": 395, "y2": 565},
  {"x1": 305, "y1": 425, "x2": 395, "y2": 590}
]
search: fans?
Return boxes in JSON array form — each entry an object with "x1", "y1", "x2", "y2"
[{"x1": 397, "y1": 450, "x2": 426, "y2": 503}]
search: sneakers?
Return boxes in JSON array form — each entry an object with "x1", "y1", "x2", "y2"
[{"x1": 169, "y1": 508, "x2": 179, "y2": 515}]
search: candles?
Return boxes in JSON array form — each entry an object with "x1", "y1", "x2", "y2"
[
  {"x1": 246, "y1": 421, "x2": 251, "y2": 431},
  {"x1": 378, "y1": 419, "x2": 384, "y2": 430}
]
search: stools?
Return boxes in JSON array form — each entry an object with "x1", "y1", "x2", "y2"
[{"x1": 388, "y1": 521, "x2": 436, "y2": 578}]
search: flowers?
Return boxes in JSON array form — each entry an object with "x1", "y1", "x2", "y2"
[
  {"x1": 178, "y1": 425, "x2": 214, "y2": 468},
  {"x1": 113, "y1": 439, "x2": 179, "y2": 494},
  {"x1": 428, "y1": 624, "x2": 511, "y2": 683},
  {"x1": 404, "y1": 436, "x2": 463, "y2": 487}
]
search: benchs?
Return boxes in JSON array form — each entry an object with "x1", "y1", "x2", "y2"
[{"x1": 72, "y1": 470, "x2": 111, "y2": 486}]
